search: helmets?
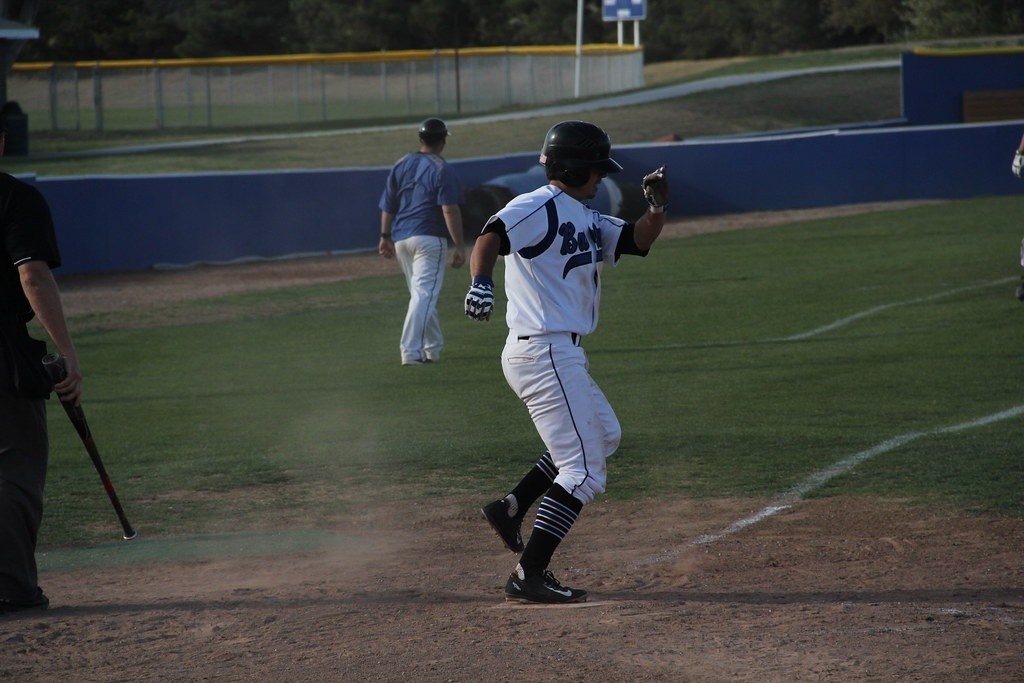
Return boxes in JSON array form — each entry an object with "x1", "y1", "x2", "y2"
[
  {"x1": 538, "y1": 120, "x2": 624, "y2": 173},
  {"x1": 418, "y1": 117, "x2": 451, "y2": 141}
]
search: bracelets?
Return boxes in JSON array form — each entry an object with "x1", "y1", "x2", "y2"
[{"x1": 381, "y1": 233, "x2": 391, "y2": 238}]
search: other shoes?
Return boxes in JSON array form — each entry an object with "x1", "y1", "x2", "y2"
[{"x1": 1015, "y1": 285, "x2": 1024, "y2": 300}]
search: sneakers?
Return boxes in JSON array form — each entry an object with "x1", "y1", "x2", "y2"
[
  {"x1": 506, "y1": 569, "x2": 589, "y2": 605},
  {"x1": 402, "y1": 358, "x2": 433, "y2": 365},
  {"x1": 425, "y1": 351, "x2": 440, "y2": 361},
  {"x1": 480, "y1": 500, "x2": 525, "y2": 554},
  {"x1": 0, "y1": 593, "x2": 49, "y2": 611}
]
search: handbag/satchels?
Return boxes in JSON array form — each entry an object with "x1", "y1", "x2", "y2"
[{"x1": 8, "y1": 334, "x2": 53, "y2": 400}]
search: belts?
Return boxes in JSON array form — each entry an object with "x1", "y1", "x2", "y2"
[{"x1": 518, "y1": 332, "x2": 583, "y2": 346}]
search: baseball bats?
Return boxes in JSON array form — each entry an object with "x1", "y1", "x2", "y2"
[{"x1": 41, "y1": 350, "x2": 136, "y2": 540}]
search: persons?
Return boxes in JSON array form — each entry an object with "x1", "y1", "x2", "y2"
[
  {"x1": 1011, "y1": 132, "x2": 1024, "y2": 303},
  {"x1": 378, "y1": 115, "x2": 468, "y2": 370},
  {"x1": 465, "y1": 120, "x2": 674, "y2": 606},
  {"x1": 1, "y1": 125, "x2": 82, "y2": 612}
]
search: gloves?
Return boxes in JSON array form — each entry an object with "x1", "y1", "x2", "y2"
[
  {"x1": 1012, "y1": 150, "x2": 1024, "y2": 179},
  {"x1": 642, "y1": 166, "x2": 669, "y2": 214},
  {"x1": 465, "y1": 274, "x2": 495, "y2": 322}
]
